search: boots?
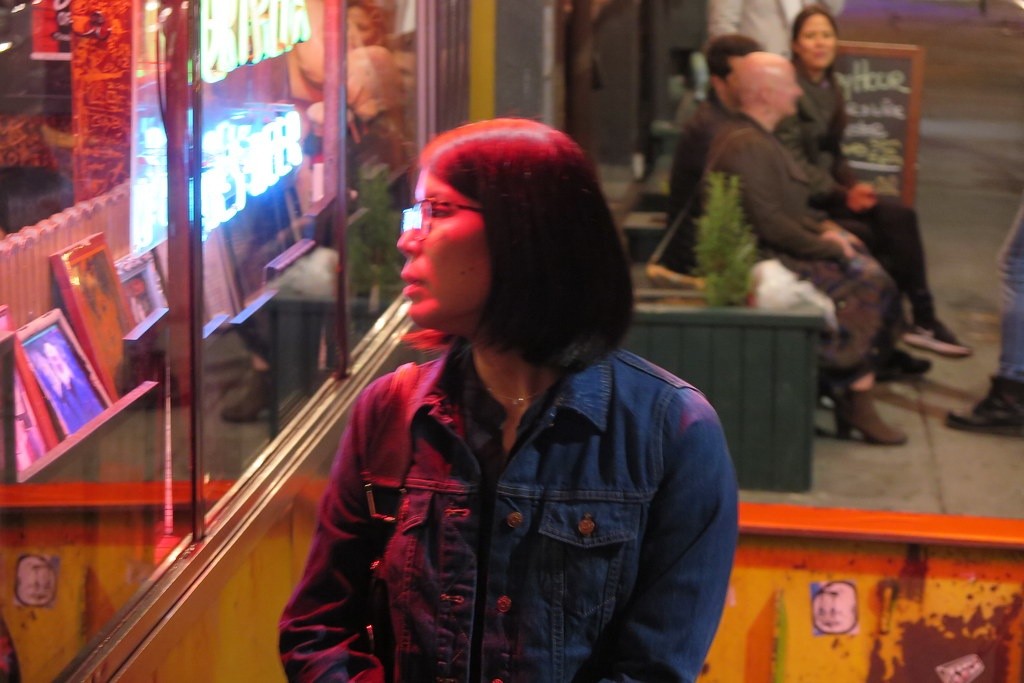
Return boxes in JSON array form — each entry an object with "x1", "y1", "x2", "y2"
[{"x1": 947, "y1": 375, "x2": 1024, "y2": 435}]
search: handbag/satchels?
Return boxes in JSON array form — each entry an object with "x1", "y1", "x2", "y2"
[
  {"x1": 645, "y1": 263, "x2": 708, "y2": 310},
  {"x1": 748, "y1": 259, "x2": 839, "y2": 334}
]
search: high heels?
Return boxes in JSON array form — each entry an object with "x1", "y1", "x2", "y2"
[
  {"x1": 834, "y1": 388, "x2": 907, "y2": 445},
  {"x1": 221, "y1": 369, "x2": 278, "y2": 422}
]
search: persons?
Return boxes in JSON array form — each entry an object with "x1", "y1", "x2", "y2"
[
  {"x1": 33, "y1": 341, "x2": 105, "y2": 429},
  {"x1": 772, "y1": 0, "x2": 974, "y2": 354},
  {"x1": 666, "y1": 36, "x2": 934, "y2": 443},
  {"x1": 278, "y1": 119, "x2": 740, "y2": 683},
  {"x1": 944, "y1": 203, "x2": 1024, "y2": 437},
  {"x1": 287, "y1": 0, "x2": 414, "y2": 218}
]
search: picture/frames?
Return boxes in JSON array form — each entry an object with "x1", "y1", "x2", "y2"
[
  {"x1": 47, "y1": 233, "x2": 139, "y2": 406},
  {"x1": 1, "y1": 304, "x2": 61, "y2": 473},
  {"x1": 114, "y1": 246, "x2": 169, "y2": 327},
  {"x1": 11, "y1": 307, "x2": 114, "y2": 443}
]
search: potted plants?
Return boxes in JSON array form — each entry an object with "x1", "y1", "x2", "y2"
[{"x1": 612, "y1": 165, "x2": 826, "y2": 495}]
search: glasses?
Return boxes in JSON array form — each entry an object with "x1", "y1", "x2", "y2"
[{"x1": 400, "y1": 198, "x2": 487, "y2": 241}]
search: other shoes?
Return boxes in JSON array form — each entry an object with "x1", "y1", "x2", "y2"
[
  {"x1": 873, "y1": 352, "x2": 930, "y2": 383},
  {"x1": 902, "y1": 321, "x2": 972, "y2": 357}
]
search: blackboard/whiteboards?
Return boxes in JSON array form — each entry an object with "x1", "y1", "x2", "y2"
[{"x1": 834, "y1": 38, "x2": 923, "y2": 209}]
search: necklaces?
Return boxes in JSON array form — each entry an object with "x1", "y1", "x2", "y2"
[{"x1": 490, "y1": 388, "x2": 544, "y2": 405}]
